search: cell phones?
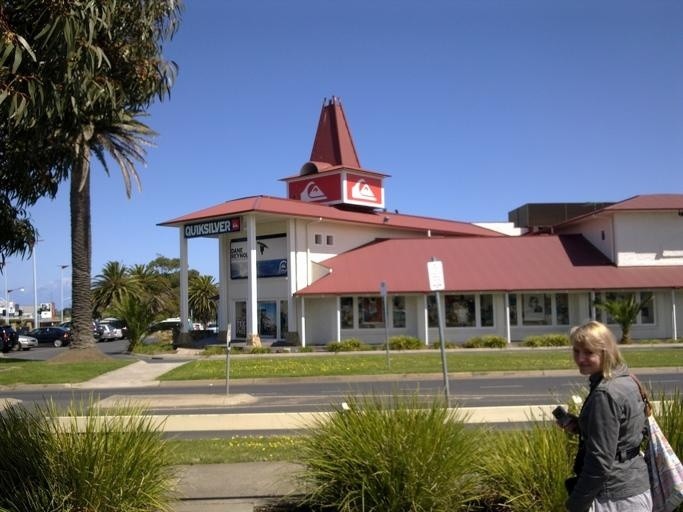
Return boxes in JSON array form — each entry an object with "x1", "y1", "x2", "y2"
[{"x1": 552, "y1": 406, "x2": 572, "y2": 427}]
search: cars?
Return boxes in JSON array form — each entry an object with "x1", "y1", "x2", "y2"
[{"x1": 1, "y1": 317, "x2": 219, "y2": 355}]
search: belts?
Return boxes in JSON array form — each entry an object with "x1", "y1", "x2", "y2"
[{"x1": 615, "y1": 447, "x2": 640, "y2": 461}]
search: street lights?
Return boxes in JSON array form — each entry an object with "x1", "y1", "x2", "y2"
[
  {"x1": 5, "y1": 286, "x2": 25, "y2": 326},
  {"x1": 60, "y1": 264, "x2": 70, "y2": 323}
]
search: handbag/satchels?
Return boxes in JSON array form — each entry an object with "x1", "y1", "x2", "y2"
[{"x1": 627, "y1": 374, "x2": 683, "y2": 512}]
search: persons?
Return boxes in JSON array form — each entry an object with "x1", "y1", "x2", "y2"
[{"x1": 554, "y1": 319, "x2": 657, "y2": 512}]
